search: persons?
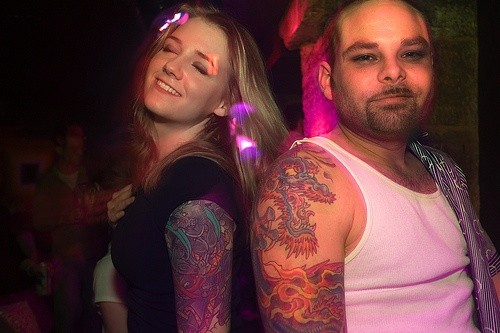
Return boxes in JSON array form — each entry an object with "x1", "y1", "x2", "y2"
[
  {"x1": 248, "y1": 0, "x2": 500, "y2": 332},
  {"x1": 0, "y1": 123, "x2": 130, "y2": 333},
  {"x1": 105, "y1": 0, "x2": 291, "y2": 332}
]
